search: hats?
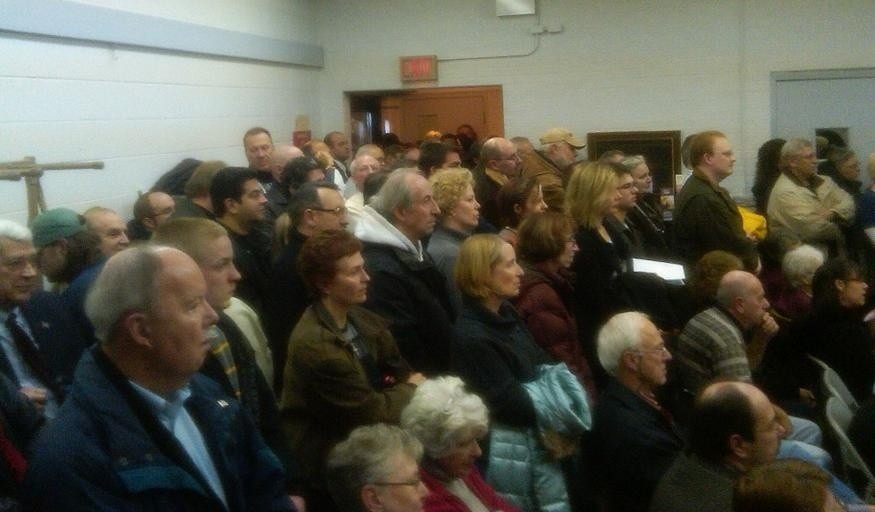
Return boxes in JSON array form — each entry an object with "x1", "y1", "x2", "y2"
[
  {"x1": 539, "y1": 127, "x2": 585, "y2": 150},
  {"x1": 30, "y1": 208, "x2": 88, "y2": 246}
]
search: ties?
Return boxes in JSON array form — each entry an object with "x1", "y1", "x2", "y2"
[{"x1": 6, "y1": 314, "x2": 64, "y2": 401}]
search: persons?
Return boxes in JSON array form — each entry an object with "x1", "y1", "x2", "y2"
[{"x1": 1, "y1": 124, "x2": 874, "y2": 511}]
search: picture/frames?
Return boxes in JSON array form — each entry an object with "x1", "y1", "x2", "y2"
[{"x1": 586, "y1": 130, "x2": 680, "y2": 199}]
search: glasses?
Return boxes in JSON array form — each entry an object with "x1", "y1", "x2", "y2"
[
  {"x1": 152, "y1": 208, "x2": 173, "y2": 220},
  {"x1": 639, "y1": 344, "x2": 665, "y2": 355},
  {"x1": 312, "y1": 206, "x2": 348, "y2": 217},
  {"x1": 374, "y1": 475, "x2": 422, "y2": 490},
  {"x1": 849, "y1": 275, "x2": 866, "y2": 283}
]
